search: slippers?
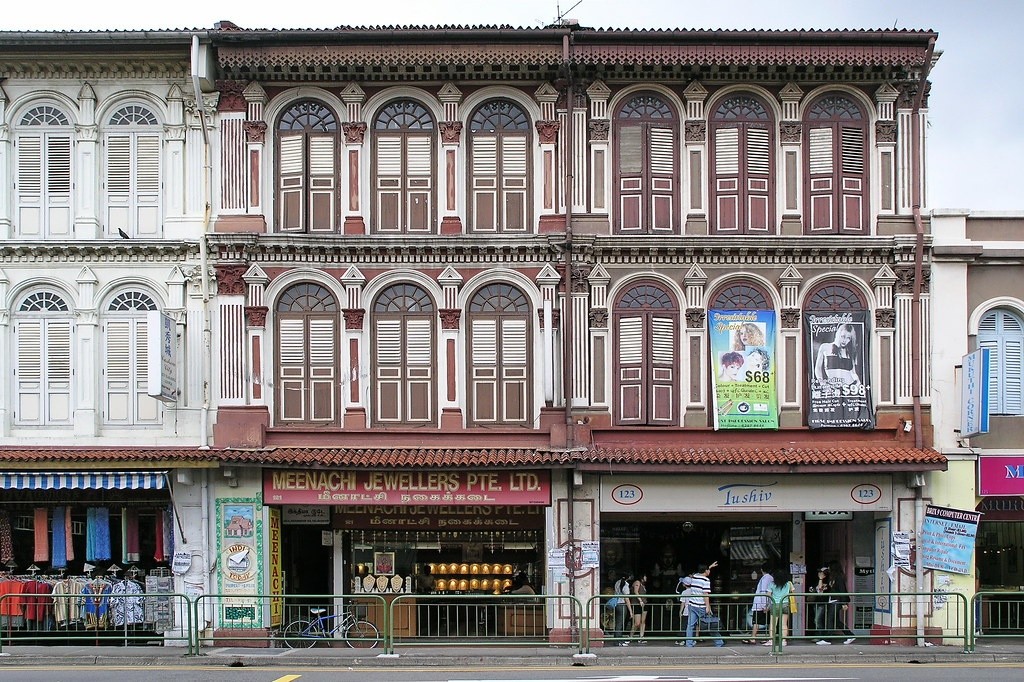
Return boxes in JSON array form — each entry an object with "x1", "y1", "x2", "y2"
[
  {"x1": 760, "y1": 639, "x2": 769, "y2": 644},
  {"x1": 742, "y1": 640, "x2": 756, "y2": 645}
]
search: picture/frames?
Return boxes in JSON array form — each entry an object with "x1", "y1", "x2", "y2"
[{"x1": 374, "y1": 552, "x2": 394, "y2": 575}]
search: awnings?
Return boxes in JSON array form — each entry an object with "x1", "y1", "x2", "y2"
[{"x1": 0, "y1": 472, "x2": 185, "y2": 541}]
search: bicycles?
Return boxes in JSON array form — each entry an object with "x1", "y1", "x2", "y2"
[{"x1": 283, "y1": 601, "x2": 380, "y2": 649}]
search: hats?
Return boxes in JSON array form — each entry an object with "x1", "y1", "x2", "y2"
[
  {"x1": 679, "y1": 577, "x2": 692, "y2": 585},
  {"x1": 817, "y1": 567, "x2": 829, "y2": 575}
]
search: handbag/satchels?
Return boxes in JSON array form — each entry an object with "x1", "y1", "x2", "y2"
[
  {"x1": 700, "y1": 612, "x2": 722, "y2": 630},
  {"x1": 605, "y1": 598, "x2": 618, "y2": 610},
  {"x1": 788, "y1": 581, "x2": 797, "y2": 613}
]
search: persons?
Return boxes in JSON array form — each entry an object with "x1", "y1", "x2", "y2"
[
  {"x1": 614, "y1": 572, "x2": 649, "y2": 646},
  {"x1": 815, "y1": 324, "x2": 865, "y2": 420},
  {"x1": 502, "y1": 572, "x2": 538, "y2": 602},
  {"x1": 742, "y1": 563, "x2": 795, "y2": 646},
  {"x1": 809, "y1": 561, "x2": 856, "y2": 645},
  {"x1": 674, "y1": 561, "x2": 727, "y2": 647},
  {"x1": 418, "y1": 566, "x2": 436, "y2": 593},
  {"x1": 719, "y1": 323, "x2": 769, "y2": 382}
]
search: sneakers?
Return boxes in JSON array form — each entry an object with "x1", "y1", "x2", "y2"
[
  {"x1": 816, "y1": 639, "x2": 831, "y2": 645},
  {"x1": 843, "y1": 638, "x2": 856, "y2": 645}
]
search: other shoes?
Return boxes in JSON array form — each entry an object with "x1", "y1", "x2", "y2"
[
  {"x1": 811, "y1": 639, "x2": 820, "y2": 643},
  {"x1": 614, "y1": 641, "x2": 630, "y2": 647},
  {"x1": 762, "y1": 640, "x2": 772, "y2": 646},
  {"x1": 692, "y1": 640, "x2": 697, "y2": 647},
  {"x1": 638, "y1": 635, "x2": 648, "y2": 644},
  {"x1": 975, "y1": 631, "x2": 983, "y2": 640},
  {"x1": 674, "y1": 640, "x2": 685, "y2": 646},
  {"x1": 781, "y1": 641, "x2": 787, "y2": 646}
]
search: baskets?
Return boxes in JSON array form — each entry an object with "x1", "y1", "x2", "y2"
[{"x1": 352, "y1": 603, "x2": 368, "y2": 619}]
